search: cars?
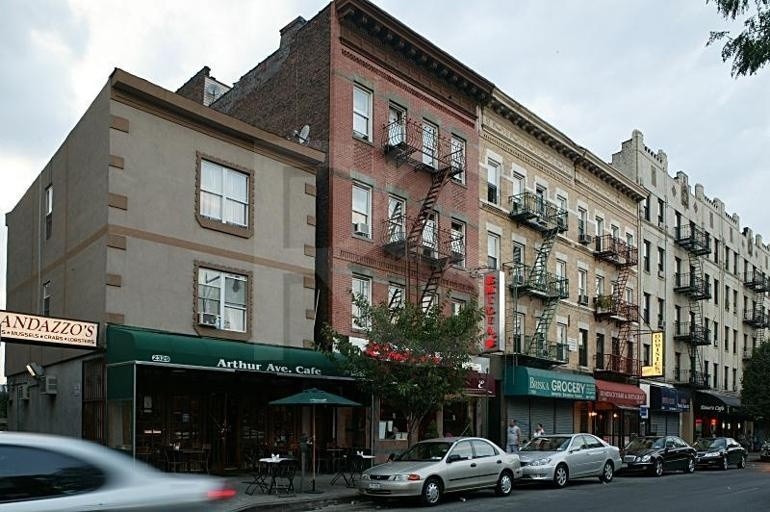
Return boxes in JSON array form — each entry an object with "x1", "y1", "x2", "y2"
[
  {"x1": 515, "y1": 433, "x2": 623, "y2": 488},
  {"x1": 620, "y1": 435, "x2": 697, "y2": 477},
  {"x1": 760, "y1": 441, "x2": 770, "y2": 460},
  {"x1": 359, "y1": 437, "x2": 520, "y2": 506},
  {"x1": 0, "y1": 431, "x2": 236, "y2": 512},
  {"x1": 692, "y1": 437, "x2": 748, "y2": 470}
]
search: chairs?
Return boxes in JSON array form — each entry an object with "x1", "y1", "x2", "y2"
[{"x1": 137, "y1": 434, "x2": 376, "y2": 496}]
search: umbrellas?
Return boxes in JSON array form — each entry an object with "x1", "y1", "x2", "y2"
[{"x1": 269, "y1": 387, "x2": 363, "y2": 490}]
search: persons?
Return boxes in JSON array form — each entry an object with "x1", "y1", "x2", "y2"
[
  {"x1": 508, "y1": 418, "x2": 522, "y2": 452},
  {"x1": 533, "y1": 423, "x2": 545, "y2": 437}
]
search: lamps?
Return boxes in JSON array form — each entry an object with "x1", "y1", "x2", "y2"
[{"x1": 24, "y1": 361, "x2": 44, "y2": 379}]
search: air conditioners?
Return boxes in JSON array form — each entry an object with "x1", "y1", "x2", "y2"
[
  {"x1": 514, "y1": 274, "x2": 523, "y2": 283},
  {"x1": 659, "y1": 223, "x2": 666, "y2": 230},
  {"x1": 355, "y1": 222, "x2": 371, "y2": 236},
  {"x1": 198, "y1": 313, "x2": 220, "y2": 329},
  {"x1": 578, "y1": 233, "x2": 592, "y2": 244},
  {"x1": 579, "y1": 294, "x2": 589, "y2": 305},
  {"x1": 15, "y1": 386, "x2": 30, "y2": 400},
  {"x1": 40, "y1": 376, "x2": 59, "y2": 395},
  {"x1": 743, "y1": 351, "x2": 751, "y2": 358},
  {"x1": 538, "y1": 349, "x2": 550, "y2": 358},
  {"x1": 658, "y1": 271, "x2": 665, "y2": 278},
  {"x1": 659, "y1": 320, "x2": 666, "y2": 327}
]
